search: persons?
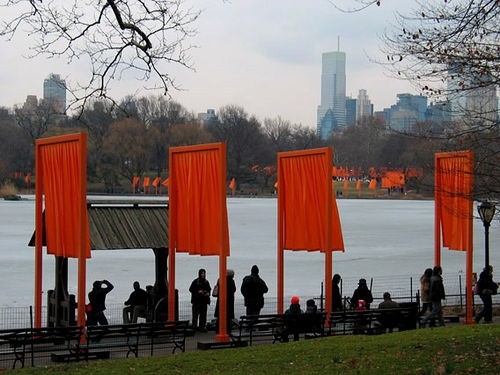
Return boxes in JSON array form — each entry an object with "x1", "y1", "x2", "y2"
[
  {"x1": 472, "y1": 265, "x2": 498, "y2": 322},
  {"x1": 188, "y1": 268, "x2": 212, "y2": 331},
  {"x1": 350, "y1": 279, "x2": 374, "y2": 334},
  {"x1": 85, "y1": 279, "x2": 115, "y2": 343},
  {"x1": 123, "y1": 280, "x2": 154, "y2": 325},
  {"x1": 285, "y1": 296, "x2": 322, "y2": 342},
  {"x1": 421, "y1": 266, "x2": 448, "y2": 327},
  {"x1": 332, "y1": 273, "x2": 343, "y2": 311},
  {"x1": 240, "y1": 265, "x2": 268, "y2": 332},
  {"x1": 336, "y1": 190, "x2": 343, "y2": 197},
  {"x1": 214, "y1": 270, "x2": 237, "y2": 336},
  {"x1": 378, "y1": 291, "x2": 400, "y2": 335}
]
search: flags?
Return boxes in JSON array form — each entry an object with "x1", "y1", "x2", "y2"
[
  {"x1": 8, "y1": 141, "x2": 94, "y2": 259},
  {"x1": 128, "y1": 149, "x2": 278, "y2": 257},
  {"x1": 439, "y1": 157, "x2": 470, "y2": 252},
  {"x1": 281, "y1": 154, "x2": 346, "y2": 253},
  {"x1": 333, "y1": 164, "x2": 425, "y2": 190}
]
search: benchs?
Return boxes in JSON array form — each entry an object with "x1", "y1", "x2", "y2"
[
  {"x1": 0, "y1": 319, "x2": 189, "y2": 370},
  {"x1": 230, "y1": 306, "x2": 420, "y2": 346}
]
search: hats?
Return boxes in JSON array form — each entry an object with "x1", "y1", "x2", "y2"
[{"x1": 291, "y1": 297, "x2": 299, "y2": 304}]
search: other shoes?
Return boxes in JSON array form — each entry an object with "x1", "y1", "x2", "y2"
[
  {"x1": 421, "y1": 322, "x2": 425, "y2": 329},
  {"x1": 474, "y1": 318, "x2": 480, "y2": 324}
]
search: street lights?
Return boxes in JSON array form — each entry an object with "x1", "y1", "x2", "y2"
[{"x1": 477, "y1": 199, "x2": 496, "y2": 266}]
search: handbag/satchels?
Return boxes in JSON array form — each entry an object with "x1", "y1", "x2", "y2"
[{"x1": 213, "y1": 279, "x2": 219, "y2": 297}]
